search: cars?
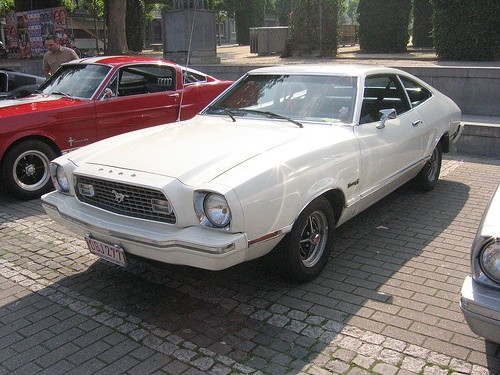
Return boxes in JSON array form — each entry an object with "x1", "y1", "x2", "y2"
[
  {"x1": 0, "y1": 70, "x2": 49, "y2": 102},
  {"x1": 0, "y1": 52, "x2": 259, "y2": 201},
  {"x1": 40, "y1": 62, "x2": 463, "y2": 284}
]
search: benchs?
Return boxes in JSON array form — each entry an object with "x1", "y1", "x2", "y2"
[{"x1": 281, "y1": 96, "x2": 405, "y2": 121}]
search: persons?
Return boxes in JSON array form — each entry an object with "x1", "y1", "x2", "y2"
[
  {"x1": 19, "y1": 30, "x2": 29, "y2": 59},
  {"x1": 42, "y1": 34, "x2": 79, "y2": 78}
]
